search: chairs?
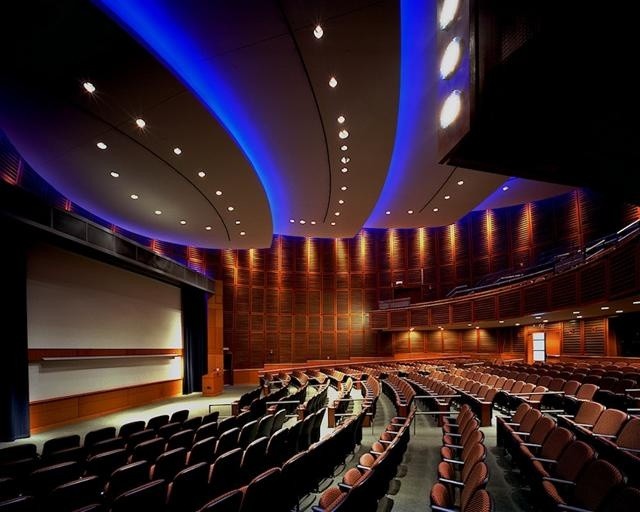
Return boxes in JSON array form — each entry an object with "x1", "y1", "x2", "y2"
[
  {"x1": 232, "y1": 356, "x2": 640, "y2": 418},
  {"x1": 1, "y1": 409, "x2": 640, "y2": 510}
]
